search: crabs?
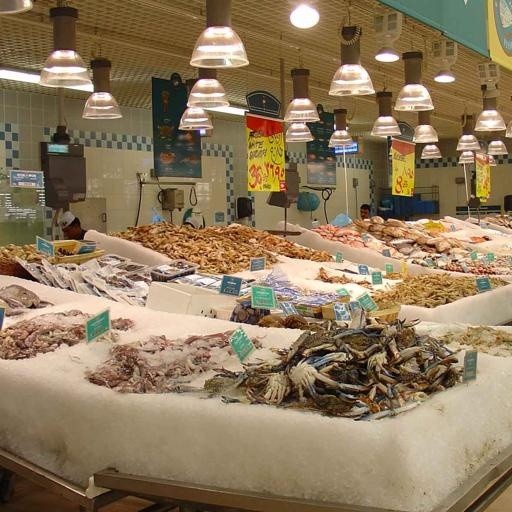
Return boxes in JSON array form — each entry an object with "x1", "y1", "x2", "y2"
[{"x1": 213, "y1": 316, "x2": 465, "y2": 421}]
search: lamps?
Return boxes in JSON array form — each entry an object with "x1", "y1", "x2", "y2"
[
  {"x1": 40, "y1": 0, "x2": 125, "y2": 123},
  {"x1": 328, "y1": 0, "x2": 511, "y2": 168},
  {"x1": 281, "y1": 45, "x2": 321, "y2": 145},
  {"x1": 177, "y1": 1, "x2": 251, "y2": 136}
]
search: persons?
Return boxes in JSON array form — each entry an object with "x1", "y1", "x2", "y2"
[
  {"x1": 58, "y1": 210, "x2": 89, "y2": 241},
  {"x1": 359, "y1": 203, "x2": 371, "y2": 220}
]
